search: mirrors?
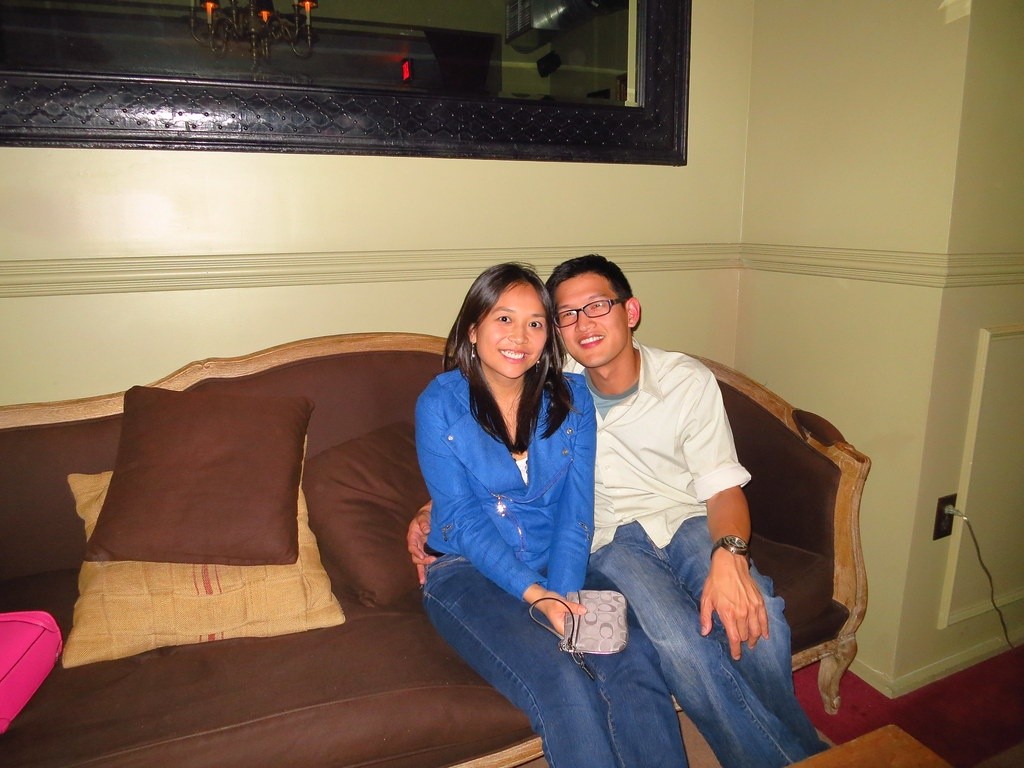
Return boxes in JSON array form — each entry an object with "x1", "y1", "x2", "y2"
[{"x1": 0, "y1": 1, "x2": 693, "y2": 168}]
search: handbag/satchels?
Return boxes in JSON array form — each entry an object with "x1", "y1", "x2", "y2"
[{"x1": 528, "y1": 589, "x2": 629, "y2": 681}]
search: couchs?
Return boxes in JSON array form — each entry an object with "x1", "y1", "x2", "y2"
[{"x1": 1, "y1": 331, "x2": 873, "y2": 768}]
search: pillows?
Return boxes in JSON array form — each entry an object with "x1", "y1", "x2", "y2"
[
  {"x1": 62, "y1": 434, "x2": 344, "y2": 669},
  {"x1": 305, "y1": 424, "x2": 433, "y2": 607},
  {"x1": 80, "y1": 377, "x2": 313, "y2": 565}
]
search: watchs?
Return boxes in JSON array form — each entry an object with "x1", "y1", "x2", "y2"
[{"x1": 711, "y1": 535, "x2": 751, "y2": 568}]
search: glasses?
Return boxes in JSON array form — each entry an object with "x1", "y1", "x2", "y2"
[{"x1": 552, "y1": 298, "x2": 624, "y2": 328}]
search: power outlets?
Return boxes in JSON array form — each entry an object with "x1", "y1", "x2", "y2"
[{"x1": 932, "y1": 492, "x2": 957, "y2": 540}]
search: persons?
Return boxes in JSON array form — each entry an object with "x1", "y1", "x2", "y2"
[
  {"x1": 415, "y1": 264, "x2": 689, "y2": 767},
  {"x1": 408, "y1": 253, "x2": 831, "y2": 768}
]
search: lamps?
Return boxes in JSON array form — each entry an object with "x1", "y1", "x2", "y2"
[{"x1": 186, "y1": 0, "x2": 315, "y2": 71}]
